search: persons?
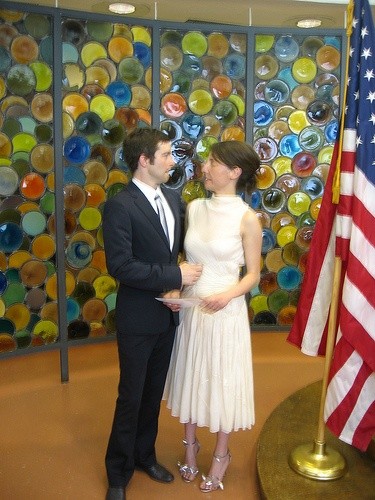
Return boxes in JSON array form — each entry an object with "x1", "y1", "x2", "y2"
[
  {"x1": 101, "y1": 127, "x2": 204, "y2": 500},
  {"x1": 162, "y1": 139, "x2": 264, "y2": 493}
]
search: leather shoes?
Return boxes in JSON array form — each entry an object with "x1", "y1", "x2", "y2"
[
  {"x1": 137, "y1": 463, "x2": 174, "y2": 483},
  {"x1": 105, "y1": 484, "x2": 125, "y2": 500}
]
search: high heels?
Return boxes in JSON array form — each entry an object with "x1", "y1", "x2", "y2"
[
  {"x1": 199, "y1": 448, "x2": 232, "y2": 492},
  {"x1": 179, "y1": 437, "x2": 201, "y2": 483}
]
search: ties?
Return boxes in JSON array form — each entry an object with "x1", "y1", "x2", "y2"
[{"x1": 154, "y1": 194, "x2": 170, "y2": 246}]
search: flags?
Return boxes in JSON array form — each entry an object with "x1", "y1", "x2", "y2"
[{"x1": 287, "y1": 0, "x2": 374, "y2": 457}]
